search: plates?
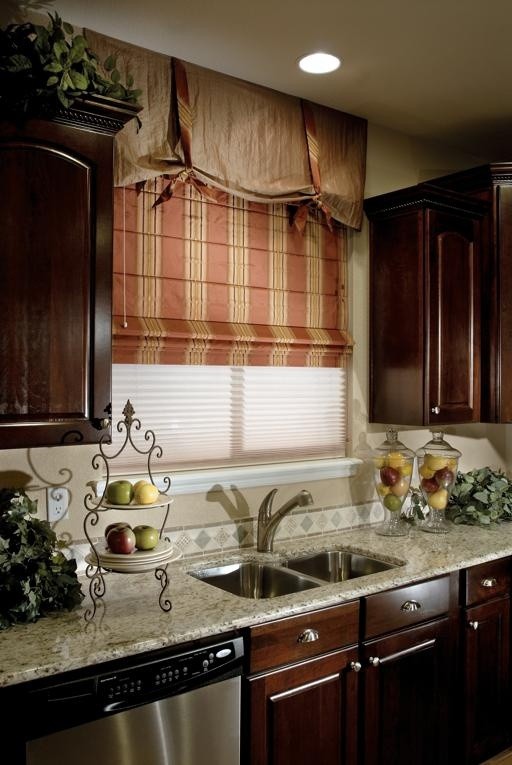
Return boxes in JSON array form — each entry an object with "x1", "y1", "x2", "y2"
[
  {"x1": 97, "y1": 493, "x2": 173, "y2": 510},
  {"x1": 84, "y1": 540, "x2": 184, "y2": 572}
]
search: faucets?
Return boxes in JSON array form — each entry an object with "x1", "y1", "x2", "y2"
[{"x1": 256, "y1": 487, "x2": 315, "y2": 553}]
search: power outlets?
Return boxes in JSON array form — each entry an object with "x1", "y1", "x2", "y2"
[{"x1": 46, "y1": 488, "x2": 71, "y2": 523}]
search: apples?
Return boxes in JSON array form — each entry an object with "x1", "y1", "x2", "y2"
[
  {"x1": 106, "y1": 479, "x2": 158, "y2": 505},
  {"x1": 420, "y1": 455, "x2": 456, "y2": 491},
  {"x1": 378, "y1": 452, "x2": 410, "y2": 511},
  {"x1": 104, "y1": 522, "x2": 160, "y2": 554}
]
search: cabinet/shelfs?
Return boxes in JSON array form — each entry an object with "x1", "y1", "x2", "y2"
[
  {"x1": 454, "y1": 556, "x2": 512, "y2": 758},
  {"x1": 245, "y1": 599, "x2": 363, "y2": 765},
  {"x1": 362, "y1": 160, "x2": 512, "y2": 427},
  {"x1": 0, "y1": 88, "x2": 141, "y2": 446},
  {"x1": 362, "y1": 574, "x2": 459, "y2": 765}
]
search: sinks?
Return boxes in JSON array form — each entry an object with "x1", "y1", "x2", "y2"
[
  {"x1": 278, "y1": 543, "x2": 410, "y2": 585},
  {"x1": 185, "y1": 560, "x2": 323, "y2": 600}
]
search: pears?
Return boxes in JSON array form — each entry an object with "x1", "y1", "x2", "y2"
[{"x1": 429, "y1": 489, "x2": 447, "y2": 509}]
